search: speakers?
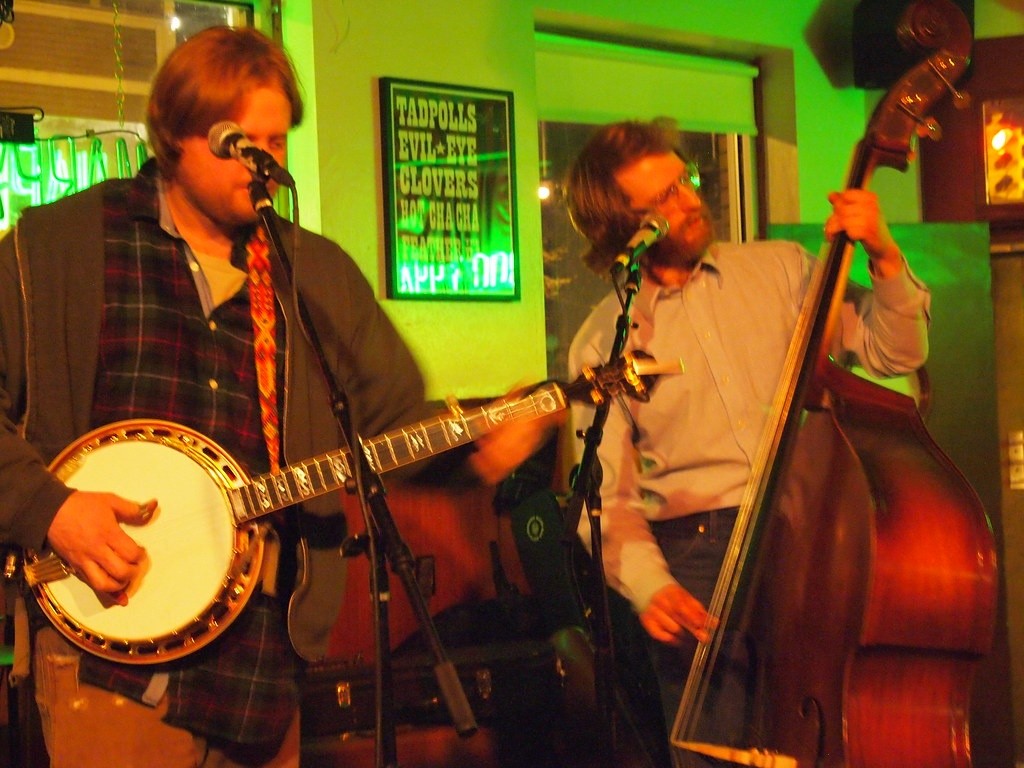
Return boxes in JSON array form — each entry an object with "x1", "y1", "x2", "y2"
[{"x1": 853, "y1": 0, "x2": 975, "y2": 91}]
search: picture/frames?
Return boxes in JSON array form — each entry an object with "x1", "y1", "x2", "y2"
[{"x1": 377, "y1": 77, "x2": 523, "y2": 304}]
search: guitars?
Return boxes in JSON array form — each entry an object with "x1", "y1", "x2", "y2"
[{"x1": 24, "y1": 348, "x2": 662, "y2": 667}]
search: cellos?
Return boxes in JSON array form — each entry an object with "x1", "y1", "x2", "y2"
[{"x1": 672, "y1": 47, "x2": 1001, "y2": 768}]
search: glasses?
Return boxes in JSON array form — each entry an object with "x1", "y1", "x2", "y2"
[{"x1": 634, "y1": 161, "x2": 701, "y2": 215}]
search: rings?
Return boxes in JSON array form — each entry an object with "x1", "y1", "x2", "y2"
[{"x1": 138, "y1": 501, "x2": 150, "y2": 524}]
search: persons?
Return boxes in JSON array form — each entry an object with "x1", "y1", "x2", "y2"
[
  {"x1": 0, "y1": 26, "x2": 568, "y2": 768},
  {"x1": 556, "y1": 115, "x2": 938, "y2": 768}
]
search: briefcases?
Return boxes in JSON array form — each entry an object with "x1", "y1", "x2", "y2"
[{"x1": 301, "y1": 639, "x2": 555, "y2": 734}]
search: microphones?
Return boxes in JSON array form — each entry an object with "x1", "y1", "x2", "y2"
[
  {"x1": 208, "y1": 121, "x2": 295, "y2": 187},
  {"x1": 610, "y1": 214, "x2": 669, "y2": 276}
]
